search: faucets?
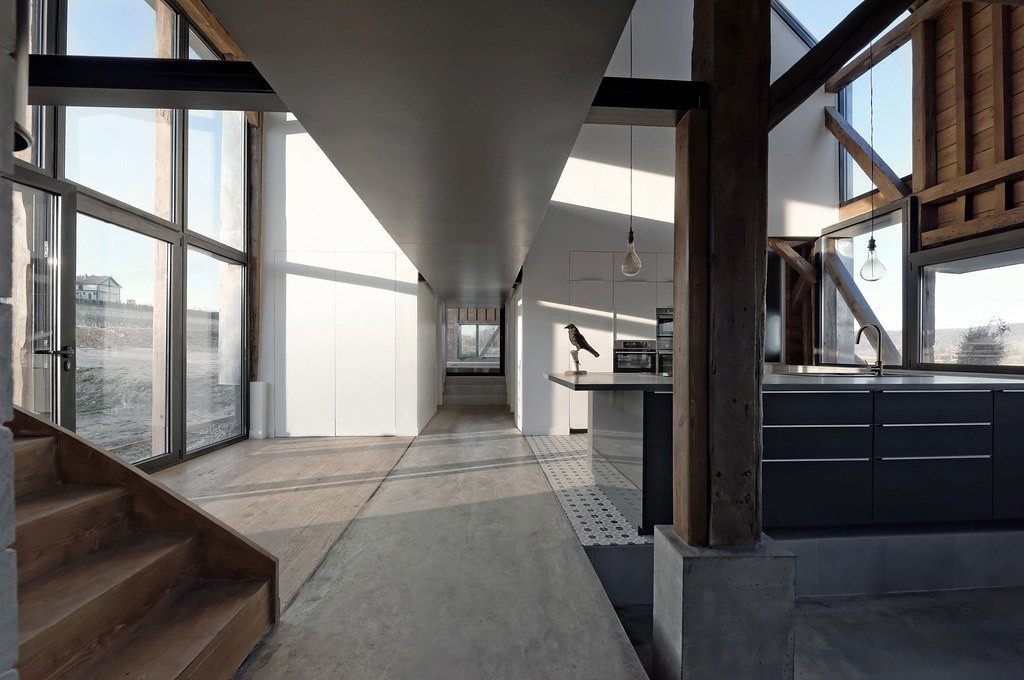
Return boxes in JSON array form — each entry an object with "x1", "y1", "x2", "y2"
[{"x1": 855, "y1": 323, "x2": 883, "y2": 377}]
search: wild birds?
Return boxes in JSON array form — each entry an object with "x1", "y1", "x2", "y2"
[{"x1": 564, "y1": 323, "x2": 600, "y2": 357}]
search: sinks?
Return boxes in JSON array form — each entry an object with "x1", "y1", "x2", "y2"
[{"x1": 770, "y1": 369, "x2": 933, "y2": 377}]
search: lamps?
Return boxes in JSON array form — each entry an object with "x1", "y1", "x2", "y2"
[
  {"x1": 860, "y1": 42, "x2": 887, "y2": 282},
  {"x1": 621, "y1": 12, "x2": 642, "y2": 276}
]
